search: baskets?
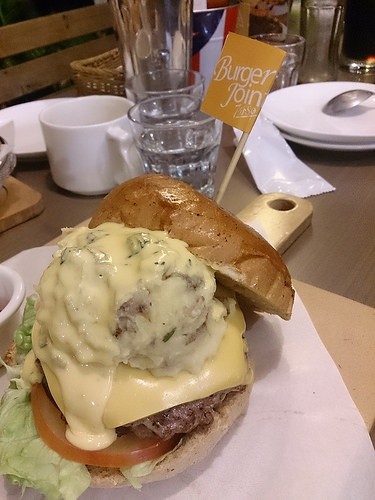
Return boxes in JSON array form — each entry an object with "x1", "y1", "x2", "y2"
[{"x1": 69, "y1": 48, "x2": 128, "y2": 97}]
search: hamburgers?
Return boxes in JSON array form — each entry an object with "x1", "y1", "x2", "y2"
[{"x1": 0, "y1": 174, "x2": 296, "y2": 499}]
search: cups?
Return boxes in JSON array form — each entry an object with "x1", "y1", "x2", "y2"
[
  {"x1": 0, "y1": 263, "x2": 26, "y2": 369},
  {"x1": 39, "y1": 95, "x2": 139, "y2": 197},
  {"x1": 124, "y1": 68, "x2": 203, "y2": 112},
  {"x1": 249, "y1": 34, "x2": 305, "y2": 93},
  {"x1": 0, "y1": 117, "x2": 16, "y2": 173},
  {"x1": 190, "y1": 0, "x2": 242, "y2": 97},
  {"x1": 133, "y1": 115, "x2": 223, "y2": 199},
  {"x1": 126, "y1": 94, "x2": 203, "y2": 140},
  {"x1": 340, "y1": 0, "x2": 375, "y2": 75},
  {"x1": 295, "y1": 0, "x2": 346, "y2": 82},
  {"x1": 245, "y1": 0, "x2": 293, "y2": 43}
]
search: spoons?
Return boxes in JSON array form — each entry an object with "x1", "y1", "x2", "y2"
[{"x1": 320, "y1": 89, "x2": 375, "y2": 113}]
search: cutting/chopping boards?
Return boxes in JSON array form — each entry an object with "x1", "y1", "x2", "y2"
[{"x1": 232, "y1": 190, "x2": 375, "y2": 500}]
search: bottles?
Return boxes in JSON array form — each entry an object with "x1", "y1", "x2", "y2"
[{"x1": 108, "y1": 0, "x2": 195, "y2": 81}]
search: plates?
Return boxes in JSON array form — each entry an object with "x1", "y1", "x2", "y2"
[
  {"x1": 0, "y1": 96, "x2": 70, "y2": 163},
  {"x1": 262, "y1": 81, "x2": 375, "y2": 151}
]
